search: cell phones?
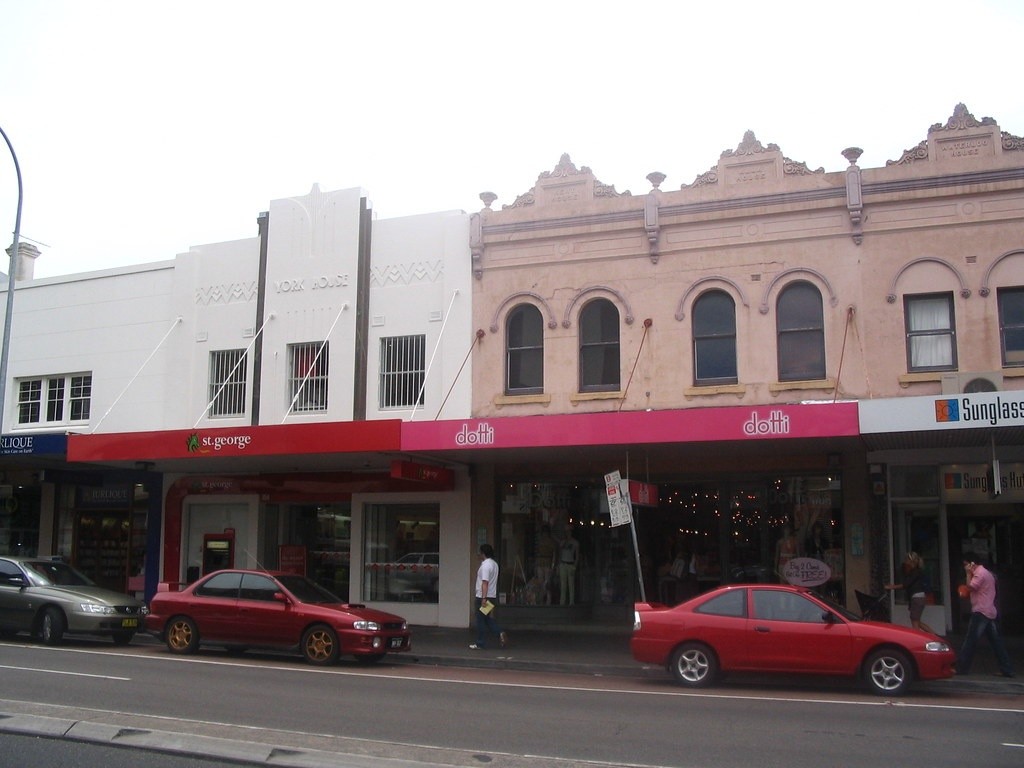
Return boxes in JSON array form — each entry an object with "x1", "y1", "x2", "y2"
[{"x1": 970, "y1": 562, "x2": 975, "y2": 567}]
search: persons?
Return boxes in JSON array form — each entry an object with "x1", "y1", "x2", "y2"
[
  {"x1": 884, "y1": 553, "x2": 936, "y2": 634},
  {"x1": 535, "y1": 521, "x2": 581, "y2": 607},
  {"x1": 949, "y1": 551, "x2": 1015, "y2": 677},
  {"x1": 673, "y1": 540, "x2": 698, "y2": 596},
  {"x1": 469, "y1": 544, "x2": 507, "y2": 649},
  {"x1": 774, "y1": 520, "x2": 835, "y2": 600}
]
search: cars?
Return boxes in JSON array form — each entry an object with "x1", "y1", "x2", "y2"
[
  {"x1": 0, "y1": 555, "x2": 149, "y2": 645},
  {"x1": 628, "y1": 584, "x2": 959, "y2": 699},
  {"x1": 140, "y1": 569, "x2": 412, "y2": 667}
]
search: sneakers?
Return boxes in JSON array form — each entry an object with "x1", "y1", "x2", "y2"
[
  {"x1": 469, "y1": 643, "x2": 485, "y2": 650},
  {"x1": 500, "y1": 633, "x2": 509, "y2": 648}
]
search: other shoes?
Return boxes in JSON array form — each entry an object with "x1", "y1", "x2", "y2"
[
  {"x1": 954, "y1": 670, "x2": 969, "y2": 675},
  {"x1": 992, "y1": 670, "x2": 1016, "y2": 678}
]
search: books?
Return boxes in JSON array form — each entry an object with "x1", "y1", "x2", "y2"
[{"x1": 479, "y1": 599, "x2": 495, "y2": 615}]
